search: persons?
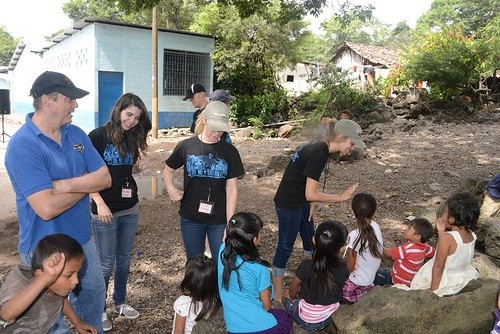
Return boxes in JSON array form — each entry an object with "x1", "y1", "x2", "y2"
[
  {"x1": 374, "y1": 217, "x2": 436, "y2": 287},
  {"x1": 163, "y1": 101, "x2": 245, "y2": 273},
  {"x1": 283, "y1": 219, "x2": 350, "y2": 332},
  {"x1": 218, "y1": 213, "x2": 294, "y2": 334},
  {"x1": 0, "y1": 233, "x2": 99, "y2": 334},
  {"x1": 340, "y1": 193, "x2": 383, "y2": 304},
  {"x1": 172, "y1": 255, "x2": 221, "y2": 334},
  {"x1": 89, "y1": 93, "x2": 153, "y2": 332},
  {"x1": 182, "y1": 83, "x2": 209, "y2": 134},
  {"x1": 3, "y1": 70, "x2": 112, "y2": 334},
  {"x1": 392, "y1": 190, "x2": 481, "y2": 297},
  {"x1": 209, "y1": 89, "x2": 237, "y2": 145},
  {"x1": 316, "y1": 109, "x2": 350, "y2": 136},
  {"x1": 273, "y1": 119, "x2": 368, "y2": 310}
]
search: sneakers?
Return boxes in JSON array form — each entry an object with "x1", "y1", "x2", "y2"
[
  {"x1": 102, "y1": 313, "x2": 112, "y2": 331},
  {"x1": 114, "y1": 304, "x2": 139, "y2": 318}
]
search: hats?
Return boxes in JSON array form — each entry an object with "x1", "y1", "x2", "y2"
[
  {"x1": 183, "y1": 84, "x2": 205, "y2": 101},
  {"x1": 209, "y1": 89, "x2": 229, "y2": 102},
  {"x1": 30, "y1": 71, "x2": 90, "y2": 99},
  {"x1": 334, "y1": 119, "x2": 367, "y2": 148},
  {"x1": 204, "y1": 101, "x2": 230, "y2": 134}
]
